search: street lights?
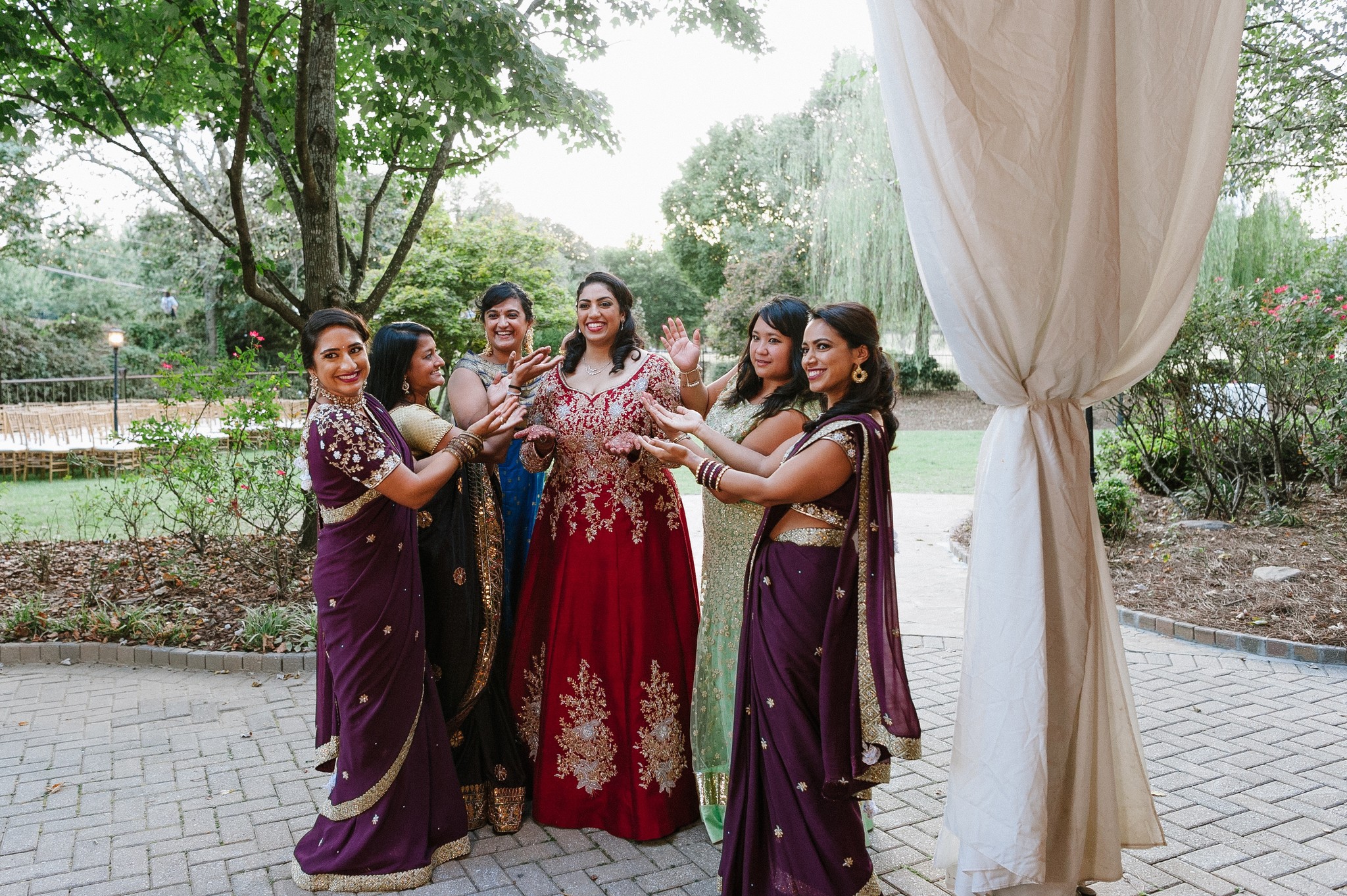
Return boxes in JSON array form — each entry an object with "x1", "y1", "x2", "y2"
[{"x1": 108, "y1": 328, "x2": 125, "y2": 433}]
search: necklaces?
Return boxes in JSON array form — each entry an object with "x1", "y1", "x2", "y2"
[{"x1": 580, "y1": 351, "x2": 613, "y2": 376}]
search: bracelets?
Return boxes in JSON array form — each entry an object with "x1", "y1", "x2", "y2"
[
  {"x1": 439, "y1": 430, "x2": 485, "y2": 469},
  {"x1": 676, "y1": 364, "x2": 702, "y2": 387},
  {"x1": 507, "y1": 390, "x2": 522, "y2": 397},
  {"x1": 670, "y1": 432, "x2": 691, "y2": 444},
  {"x1": 695, "y1": 457, "x2": 732, "y2": 492},
  {"x1": 507, "y1": 384, "x2": 521, "y2": 392}
]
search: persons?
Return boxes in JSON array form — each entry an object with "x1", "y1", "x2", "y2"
[
  {"x1": 290, "y1": 305, "x2": 519, "y2": 893},
  {"x1": 632, "y1": 302, "x2": 901, "y2": 896},
  {"x1": 658, "y1": 294, "x2": 879, "y2": 846},
  {"x1": 446, "y1": 279, "x2": 553, "y2": 618},
  {"x1": 364, "y1": 320, "x2": 568, "y2": 836},
  {"x1": 162, "y1": 291, "x2": 178, "y2": 322},
  {"x1": 511, "y1": 272, "x2": 703, "y2": 842}
]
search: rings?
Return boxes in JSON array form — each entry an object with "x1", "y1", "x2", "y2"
[
  {"x1": 500, "y1": 416, "x2": 507, "y2": 425},
  {"x1": 538, "y1": 436, "x2": 548, "y2": 443},
  {"x1": 675, "y1": 337, "x2": 681, "y2": 342}
]
